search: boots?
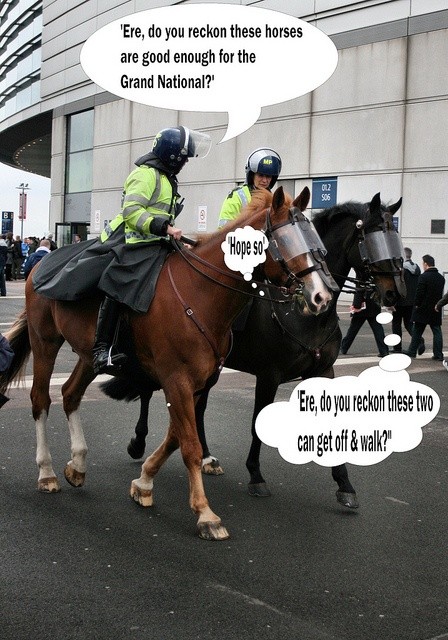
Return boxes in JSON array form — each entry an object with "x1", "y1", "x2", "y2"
[{"x1": 93, "y1": 296, "x2": 127, "y2": 373}]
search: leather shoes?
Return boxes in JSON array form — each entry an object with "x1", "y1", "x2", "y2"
[{"x1": 401, "y1": 349, "x2": 417, "y2": 357}]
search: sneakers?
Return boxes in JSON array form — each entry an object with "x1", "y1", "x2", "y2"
[
  {"x1": 417, "y1": 336, "x2": 425, "y2": 354},
  {"x1": 442, "y1": 359, "x2": 448, "y2": 370}
]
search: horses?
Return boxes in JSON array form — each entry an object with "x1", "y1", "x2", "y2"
[
  {"x1": 100, "y1": 192, "x2": 409, "y2": 510},
  {"x1": 0, "y1": 185, "x2": 341, "y2": 543}
]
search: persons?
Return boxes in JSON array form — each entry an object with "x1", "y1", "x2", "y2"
[
  {"x1": 340, "y1": 252, "x2": 388, "y2": 361},
  {"x1": 1, "y1": 229, "x2": 59, "y2": 296},
  {"x1": 434, "y1": 279, "x2": 448, "y2": 358},
  {"x1": 215, "y1": 149, "x2": 280, "y2": 233},
  {"x1": 411, "y1": 253, "x2": 444, "y2": 367},
  {"x1": 69, "y1": 231, "x2": 82, "y2": 243},
  {"x1": 91, "y1": 125, "x2": 195, "y2": 374},
  {"x1": 386, "y1": 244, "x2": 428, "y2": 363}
]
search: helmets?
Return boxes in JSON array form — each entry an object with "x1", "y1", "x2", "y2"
[
  {"x1": 246, "y1": 147, "x2": 284, "y2": 189},
  {"x1": 152, "y1": 126, "x2": 212, "y2": 170}
]
